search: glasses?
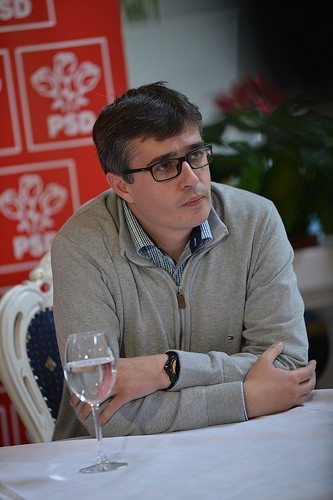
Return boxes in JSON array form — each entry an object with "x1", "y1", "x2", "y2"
[{"x1": 120, "y1": 143, "x2": 213, "y2": 182}]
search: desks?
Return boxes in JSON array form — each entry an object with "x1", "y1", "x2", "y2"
[
  {"x1": 293, "y1": 247, "x2": 333, "y2": 311},
  {"x1": 0, "y1": 387, "x2": 333, "y2": 500}
]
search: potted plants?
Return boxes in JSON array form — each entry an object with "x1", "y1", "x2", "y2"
[{"x1": 200, "y1": 76, "x2": 333, "y2": 253}]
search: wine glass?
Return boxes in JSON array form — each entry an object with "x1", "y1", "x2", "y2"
[{"x1": 63, "y1": 330, "x2": 128, "y2": 474}]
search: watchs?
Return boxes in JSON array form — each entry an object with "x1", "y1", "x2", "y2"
[{"x1": 162, "y1": 350, "x2": 180, "y2": 391}]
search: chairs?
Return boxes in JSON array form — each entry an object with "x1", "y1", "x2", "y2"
[{"x1": 0, "y1": 249, "x2": 65, "y2": 443}]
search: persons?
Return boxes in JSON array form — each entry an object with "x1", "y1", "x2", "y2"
[{"x1": 51, "y1": 79, "x2": 317, "y2": 443}]
